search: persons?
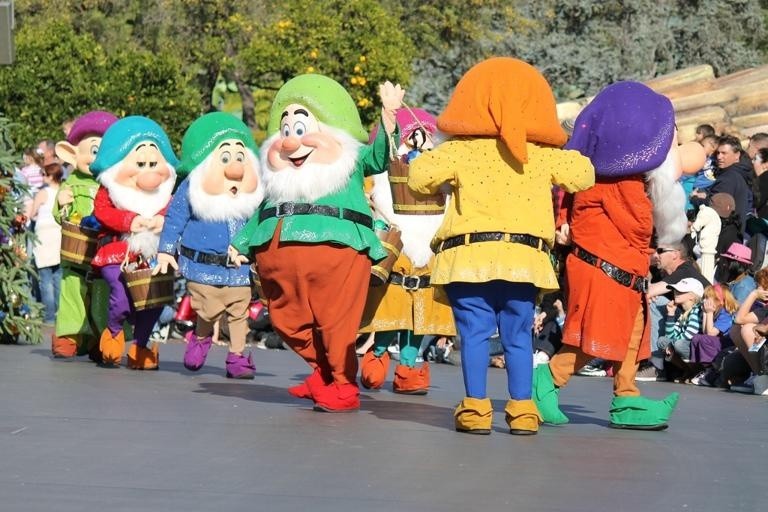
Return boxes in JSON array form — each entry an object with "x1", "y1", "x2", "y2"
[
  {"x1": 16, "y1": 125, "x2": 768, "y2": 395},
  {"x1": 154, "y1": 112, "x2": 265, "y2": 380},
  {"x1": 357, "y1": 105, "x2": 458, "y2": 396},
  {"x1": 227, "y1": 74, "x2": 404, "y2": 415},
  {"x1": 54, "y1": 112, "x2": 132, "y2": 360},
  {"x1": 89, "y1": 115, "x2": 181, "y2": 370},
  {"x1": 409, "y1": 57, "x2": 595, "y2": 432},
  {"x1": 531, "y1": 80, "x2": 705, "y2": 432}
]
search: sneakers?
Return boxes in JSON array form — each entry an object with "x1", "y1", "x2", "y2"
[
  {"x1": 387, "y1": 343, "x2": 400, "y2": 354},
  {"x1": 576, "y1": 364, "x2": 607, "y2": 377},
  {"x1": 634, "y1": 362, "x2": 768, "y2": 397},
  {"x1": 171, "y1": 325, "x2": 190, "y2": 339},
  {"x1": 430, "y1": 345, "x2": 446, "y2": 363}
]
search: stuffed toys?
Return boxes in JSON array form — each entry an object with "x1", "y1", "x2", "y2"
[{"x1": 52, "y1": 54, "x2": 706, "y2": 434}]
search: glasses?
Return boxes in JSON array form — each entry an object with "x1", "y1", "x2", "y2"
[{"x1": 656, "y1": 247, "x2": 677, "y2": 254}]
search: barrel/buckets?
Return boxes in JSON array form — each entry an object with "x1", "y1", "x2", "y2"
[
  {"x1": 59, "y1": 201, "x2": 99, "y2": 267},
  {"x1": 119, "y1": 231, "x2": 175, "y2": 312},
  {"x1": 248, "y1": 263, "x2": 270, "y2": 307},
  {"x1": 367, "y1": 198, "x2": 404, "y2": 289},
  {"x1": 381, "y1": 99, "x2": 446, "y2": 215}
]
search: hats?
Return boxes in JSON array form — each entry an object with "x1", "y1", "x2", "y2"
[
  {"x1": 709, "y1": 192, "x2": 736, "y2": 219},
  {"x1": 720, "y1": 242, "x2": 753, "y2": 265},
  {"x1": 666, "y1": 277, "x2": 704, "y2": 298}
]
